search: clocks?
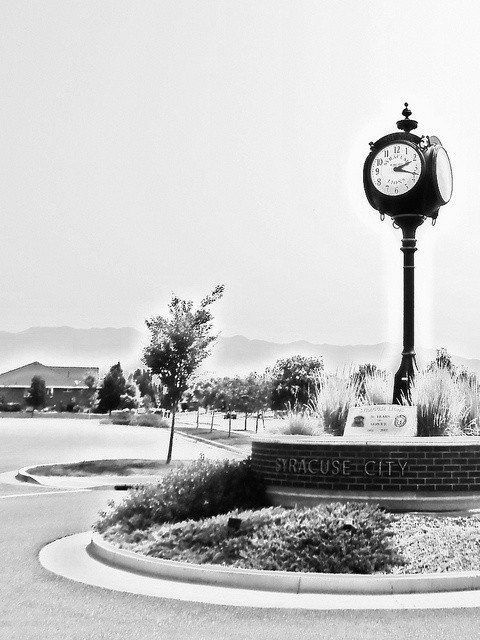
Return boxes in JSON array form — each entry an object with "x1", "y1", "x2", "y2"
[
  {"x1": 436, "y1": 148, "x2": 453, "y2": 203},
  {"x1": 369, "y1": 143, "x2": 422, "y2": 197}
]
